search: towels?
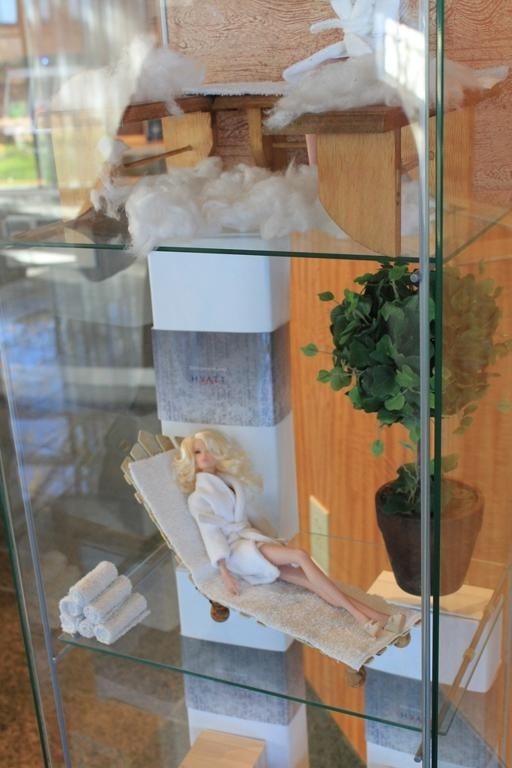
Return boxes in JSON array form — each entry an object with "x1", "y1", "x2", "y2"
[{"x1": 58, "y1": 555, "x2": 154, "y2": 648}]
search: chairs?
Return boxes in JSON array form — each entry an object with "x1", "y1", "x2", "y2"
[{"x1": 120, "y1": 426, "x2": 424, "y2": 692}]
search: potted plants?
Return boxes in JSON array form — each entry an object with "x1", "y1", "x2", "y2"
[{"x1": 301, "y1": 262, "x2": 512, "y2": 599}]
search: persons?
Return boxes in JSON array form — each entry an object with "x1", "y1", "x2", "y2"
[{"x1": 172, "y1": 429, "x2": 424, "y2": 646}]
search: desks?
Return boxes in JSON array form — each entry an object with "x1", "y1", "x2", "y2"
[
  {"x1": 286, "y1": 83, "x2": 500, "y2": 257},
  {"x1": 70, "y1": 90, "x2": 282, "y2": 211}
]
